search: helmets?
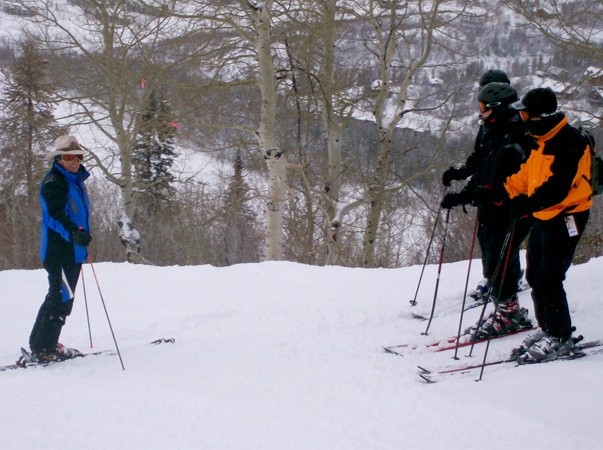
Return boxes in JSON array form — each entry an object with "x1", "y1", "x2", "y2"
[
  {"x1": 477, "y1": 82, "x2": 520, "y2": 102},
  {"x1": 478, "y1": 69, "x2": 510, "y2": 91}
]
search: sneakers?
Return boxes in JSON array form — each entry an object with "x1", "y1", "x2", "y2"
[
  {"x1": 29, "y1": 344, "x2": 72, "y2": 362},
  {"x1": 471, "y1": 297, "x2": 520, "y2": 339},
  {"x1": 521, "y1": 327, "x2": 572, "y2": 360},
  {"x1": 470, "y1": 284, "x2": 489, "y2": 302}
]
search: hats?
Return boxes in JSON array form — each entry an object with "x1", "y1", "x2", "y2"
[
  {"x1": 511, "y1": 87, "x2": 557, "y2": 117},
  {"x1": 43, "y1": 136, "x2": 87, "y2": 161}
]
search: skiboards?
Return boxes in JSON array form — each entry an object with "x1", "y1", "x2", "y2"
[
  {"x1": 416, "y1": 339, "x2": 603, "y2": 383},
  {"x1": 0, "y1": 338, "x2": 175, "y2": 371},
  {"x1": 382, "y1": 325, "x2": 541, "y2": 356},
  {"x1": 409, "y1": 281, "x2": 531, "y2": 320}
]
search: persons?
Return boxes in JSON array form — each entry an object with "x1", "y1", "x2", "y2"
[
  {"x1": 475, "y1": 85, "x2": 592, "y2": 365},
  {"x1": 28, "y1": 134, "x2": 91, "y2": 361},
  {"x1": 442, "y1": 81, "x2": 527, "y2": 341},
  {"x1": 441, "y1": 68, "x2": 524, "y2": 299}
]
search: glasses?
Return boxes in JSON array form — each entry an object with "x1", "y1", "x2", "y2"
[
  {"x1": 54, "y1": 155, "x2": 83, "y2": 163},
  {"x1": 478, "y1": 100, "x2": 488, "y2": 113},
  {"x1": 518, "y1": 111, "x2": 530, "y2": 122}
]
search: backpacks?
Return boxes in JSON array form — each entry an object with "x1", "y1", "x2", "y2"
[{"x1": 578, "y1": 125, "x2": 603, "y2": 197}]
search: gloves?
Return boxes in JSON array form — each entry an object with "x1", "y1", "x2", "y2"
[
  {"x1": 72, "y1": 229, "x2": 92, "y2": 247},
  {"x1": 442, "y1": 165, "x2": 465, "y2": 187},
  {"x1": 441, "y1": 191, "x2": 466, "y2": 210}
]
